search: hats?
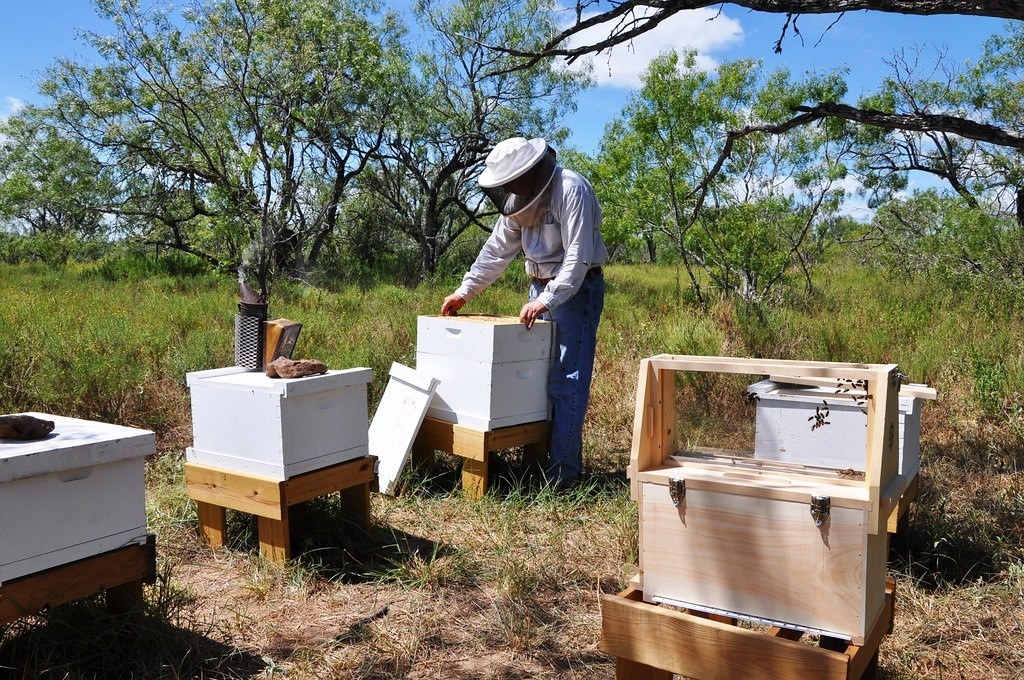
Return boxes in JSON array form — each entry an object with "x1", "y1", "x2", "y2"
[{"x1": 478, "y1": 137, "x2": 558, "y2": 219}]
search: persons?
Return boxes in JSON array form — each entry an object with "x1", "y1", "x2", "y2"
[{"x1": 441, "y1": 136, "x2": 604, "y2": 489}]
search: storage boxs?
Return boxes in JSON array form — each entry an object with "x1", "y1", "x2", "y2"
[
  {"x1": 186, "y1": 365, "x2": 373, "y2": 481},
  {"x1": 368, "y1": 314, "x2": 557, "y2": 498},
  {"x1": 748, "y1": 378, "x2": 928, "y2": 498},
  {"x1": 0, "y1": 410, "x2": 156, "y2": 587}
]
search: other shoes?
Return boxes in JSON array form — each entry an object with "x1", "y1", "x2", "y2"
[{"x1": 551, "y1": 477, "x2": 577, "y2": 503}]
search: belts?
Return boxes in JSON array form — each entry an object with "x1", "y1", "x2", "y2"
[{"x1": 534, "y1": 266, "x2": 602, "y2": 286}]
